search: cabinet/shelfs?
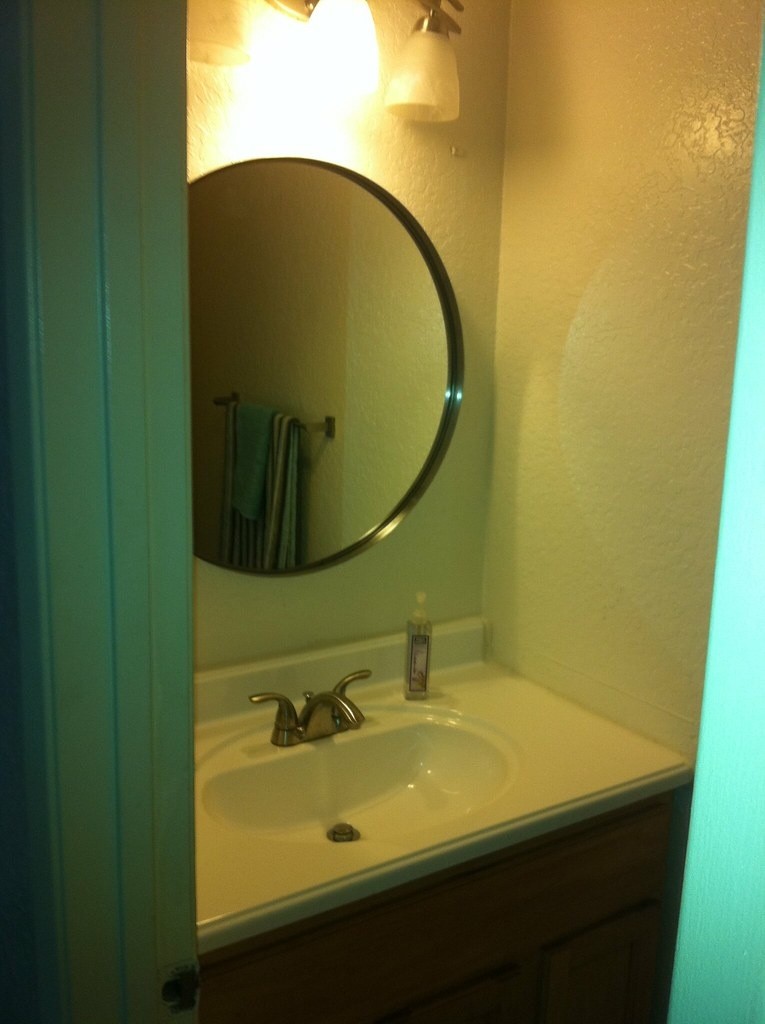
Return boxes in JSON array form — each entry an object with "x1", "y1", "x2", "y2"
[{"x1": 199, "y1": 799, "x2": 674, "y2": 1024}]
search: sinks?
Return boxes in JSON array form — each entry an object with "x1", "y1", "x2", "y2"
[{"x1": 195, "y1": 701, "x2": 533, "y2": 850}]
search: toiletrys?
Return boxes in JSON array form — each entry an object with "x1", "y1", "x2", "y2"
[{"x1": 403, "y1": 591, "x2": 434, "y2": 701}]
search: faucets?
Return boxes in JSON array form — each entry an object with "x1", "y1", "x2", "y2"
[{"x1": 298, "y1": 691, "x2": 366, "y2": 740}]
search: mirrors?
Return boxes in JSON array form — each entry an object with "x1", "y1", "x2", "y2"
[{"x1": 192, "y1": 153, "x2": 466, "y2": 576}]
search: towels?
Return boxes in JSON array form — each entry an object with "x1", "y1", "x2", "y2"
[{"x1": 218, "y1": 398, "x2": 301, "y2": 576}]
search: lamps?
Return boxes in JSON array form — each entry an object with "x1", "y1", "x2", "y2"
[
  {"x1": 383, "y1": 0, "x2": 470, "y2": 127},
  {"x1": 271, "y1": 0, "x2": 382, "y2": 90}
]
